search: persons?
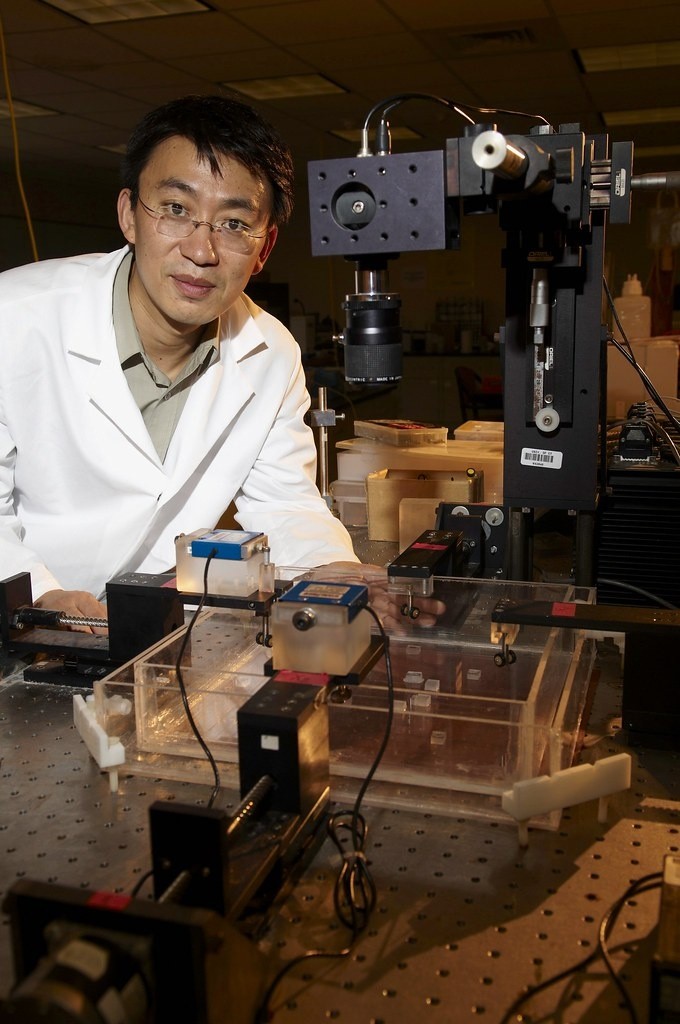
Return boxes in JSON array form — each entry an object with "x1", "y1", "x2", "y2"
[{"x1": 0, "y1": 93, "x2": 443, "y2": 639}]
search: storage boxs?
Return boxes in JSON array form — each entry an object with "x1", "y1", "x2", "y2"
[{"x1": 89, "y1": 565, "x2": 603, "y2": 832}]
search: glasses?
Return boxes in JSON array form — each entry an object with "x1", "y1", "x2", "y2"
[{"x1": 134, "y1": 192, "x2": 272, "y2": 256}]
